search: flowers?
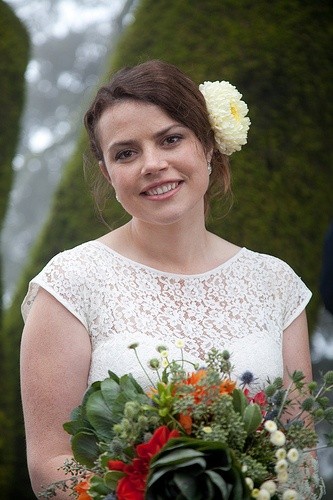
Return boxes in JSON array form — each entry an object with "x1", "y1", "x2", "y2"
[
  {"x1": 197, "y1": 79, "x2": 253, "y2": 157},
  {"x1": 33, "y1": 331, "x2": 333, "y2": 500}
]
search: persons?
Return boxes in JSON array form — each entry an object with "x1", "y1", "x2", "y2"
[{"x1": 18, "y1": 58, "x2": 323, "y2": 500}]
